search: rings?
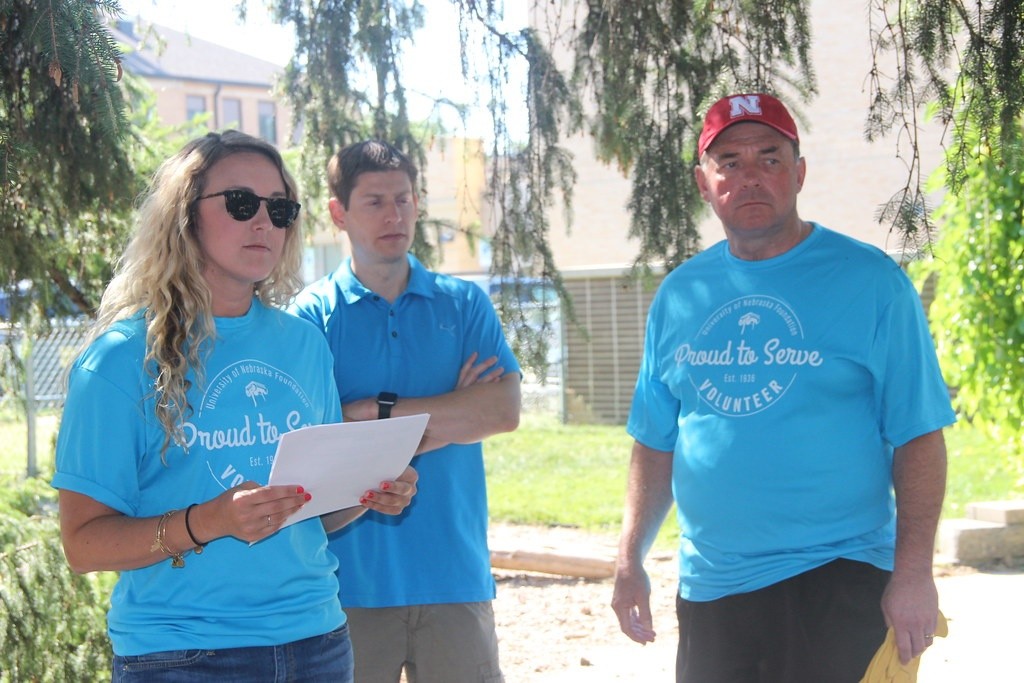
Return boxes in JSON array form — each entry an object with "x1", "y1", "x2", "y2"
[
  {"x1": 924, "y1": 634, "x2": 934, "y2": 638},
  {"x1": 266, "y1": 515, "x2": 271, "y2": 527}
]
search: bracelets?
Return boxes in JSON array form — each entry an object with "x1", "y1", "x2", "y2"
[
  {"x1": 184, "y1": 504, "x2": 208, "y2": 553},
  {"x1": 155, "y1": 509, "x2": 185, "y2": 567}
]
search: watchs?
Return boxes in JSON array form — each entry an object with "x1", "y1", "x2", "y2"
[{"x1": 376, "y1": 390, "x2": 397, "y2": 420}]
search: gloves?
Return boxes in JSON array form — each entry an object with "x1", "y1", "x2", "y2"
[{"x1": 859, "y1": 612, "x2": 948, "y2": 683}]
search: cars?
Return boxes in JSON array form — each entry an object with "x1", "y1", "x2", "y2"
[{"x1": 0, "y1": 275, "x2": 87, "y2": 321}]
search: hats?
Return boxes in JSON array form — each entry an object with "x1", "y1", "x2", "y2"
[{"x1": 698, "y1": 93, "x2": 800, "y2": 155}]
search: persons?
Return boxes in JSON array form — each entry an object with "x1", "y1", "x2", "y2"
[
  {"x1": 50, "y1": 130, "x2": 419, "y2": 683},
  {"x1": 611, "y1": 93, "x2": 958, "y2": 683},
  {"x1": 284, "y1": 139, "x2": 522, "y2": 683}
]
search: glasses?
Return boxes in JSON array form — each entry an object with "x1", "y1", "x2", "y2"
[{"x1": 195, "y1": 189, "x2": 302, "y2": 227}]
